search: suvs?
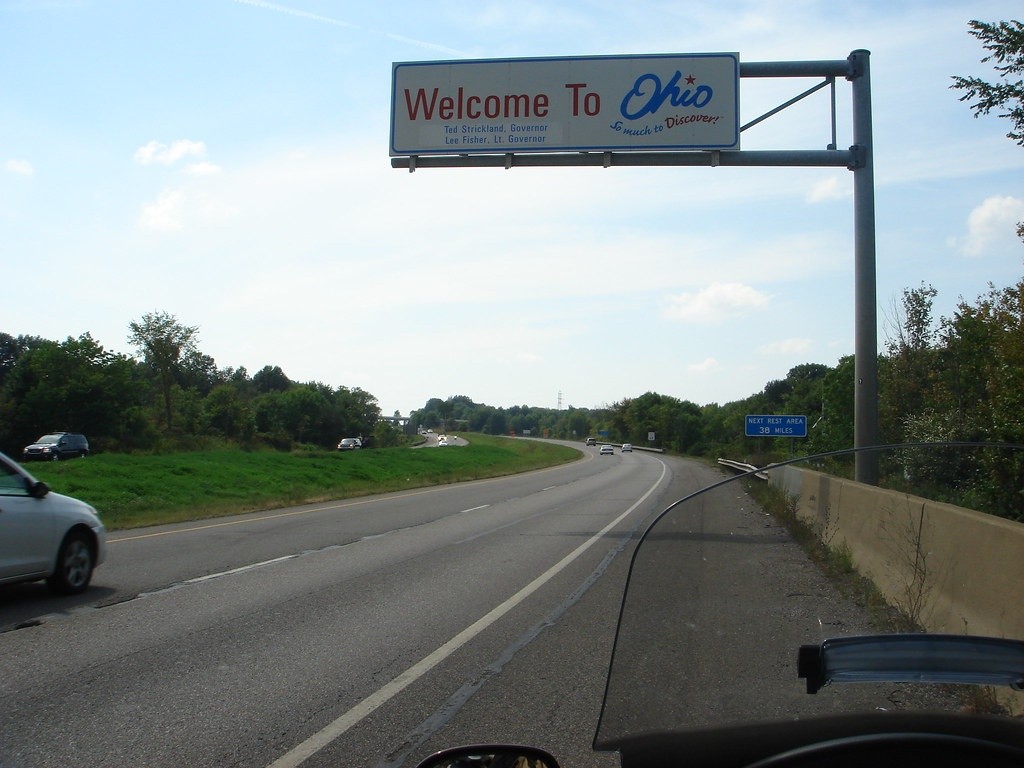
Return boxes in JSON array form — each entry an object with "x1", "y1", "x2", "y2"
[
  {"x1": 337, "y1": 438, "x2": 362, "y2": 451},
  {"x1": 586, "y1": 438, "x2": 596, "y2": 446},
  {"x1": 23, "y1": 432, "x2": 90, "y2": 464}
]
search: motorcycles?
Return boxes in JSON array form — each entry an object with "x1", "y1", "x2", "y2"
[{"x1": 415, "y1": 442, "x2": 1023, "y2": 768}]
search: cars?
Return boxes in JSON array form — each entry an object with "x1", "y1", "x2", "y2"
[
  {"x1": 0, "y1": 451, "x2": 107, "y2": 601},
  {"x1": 427, "y1": 428, "x2": 433, "y2": 433},
  {"x1": 621, "y1": 444, "x2": 632, "y2": 453},
  {"x1": 438, "y1": 435, "x2": 447, "y2": 440},
  {"x1": 422, "y1": 430, "x2": 427, "y2": 434},
  {"x1": 600, "y1": 444, "x2": 614, "y2": 455},
  {"x1": 438, "y1": 440, "x2": 448, "y2": 447}
]
